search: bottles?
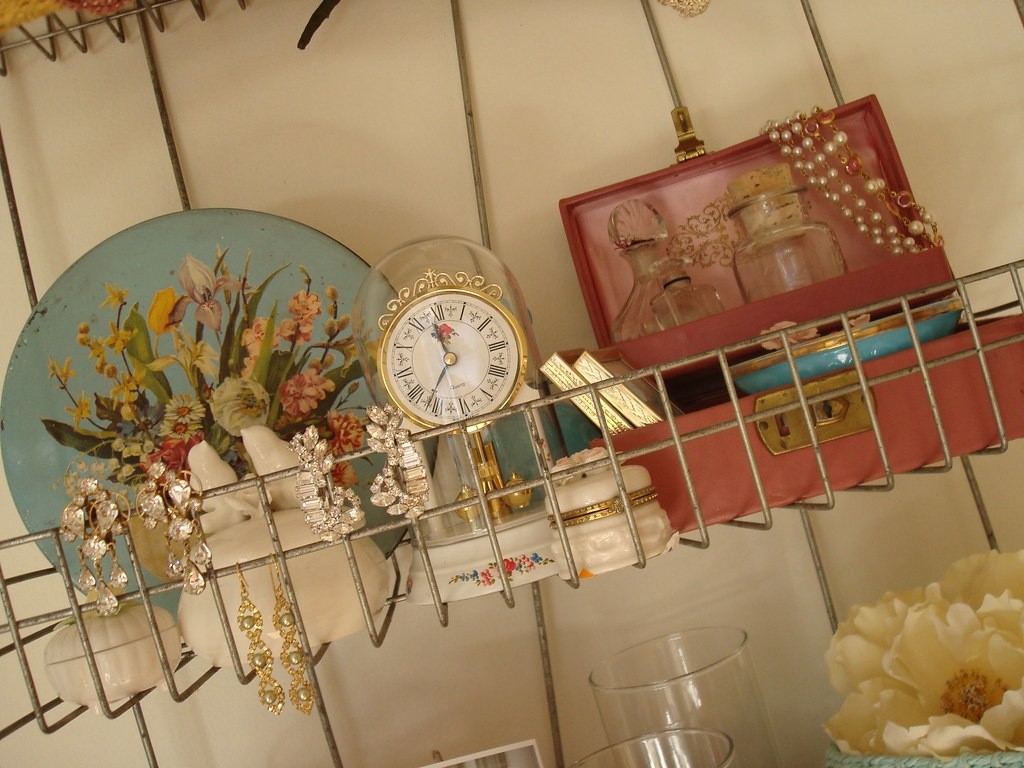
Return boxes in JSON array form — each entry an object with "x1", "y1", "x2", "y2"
[
  {"x1": 606, "y1": 199, "x2": 674, "y2": 344},
  {"x1": 648, "y1": 255, "x2": 726, "y2": 330},
  {"x1": 725, "y1": 163, "x2": 849, "y2": 305}
]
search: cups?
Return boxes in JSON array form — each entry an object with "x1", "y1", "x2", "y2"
[
  {"x1": 572, "y1": 726, "x2": 741, "y2": 768},
  {"x1": 587, "y1": 626, "x2": 779, "y2": 768}
]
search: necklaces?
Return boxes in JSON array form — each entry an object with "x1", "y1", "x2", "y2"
[{"x1": 760, "y1": 106, "x2": 945, "y2": 256}]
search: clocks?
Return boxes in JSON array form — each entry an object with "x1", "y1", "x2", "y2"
[{"x1": 353, "y1": 235, "x2": 561, "y2": 604}]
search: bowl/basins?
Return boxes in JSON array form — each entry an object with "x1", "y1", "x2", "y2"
[{"x1": 41, "y1": 602, "x2": 183, "y2": 716}]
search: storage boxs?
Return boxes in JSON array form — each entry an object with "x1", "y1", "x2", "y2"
[{"x1": 558, "y1": 94, "x2": 1024, "y2": 536}]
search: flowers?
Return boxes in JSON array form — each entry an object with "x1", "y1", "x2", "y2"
[
  {"x1": 25, "y1": 240, "x2": 377, "y2": 502},
  {"x1": 823, "y1": 550, "x2": 1023, "y2": 758}
]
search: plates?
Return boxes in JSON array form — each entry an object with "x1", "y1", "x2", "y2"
[{"x1": 726, "y1": 297, "x2": 966, "y2": 395}]
